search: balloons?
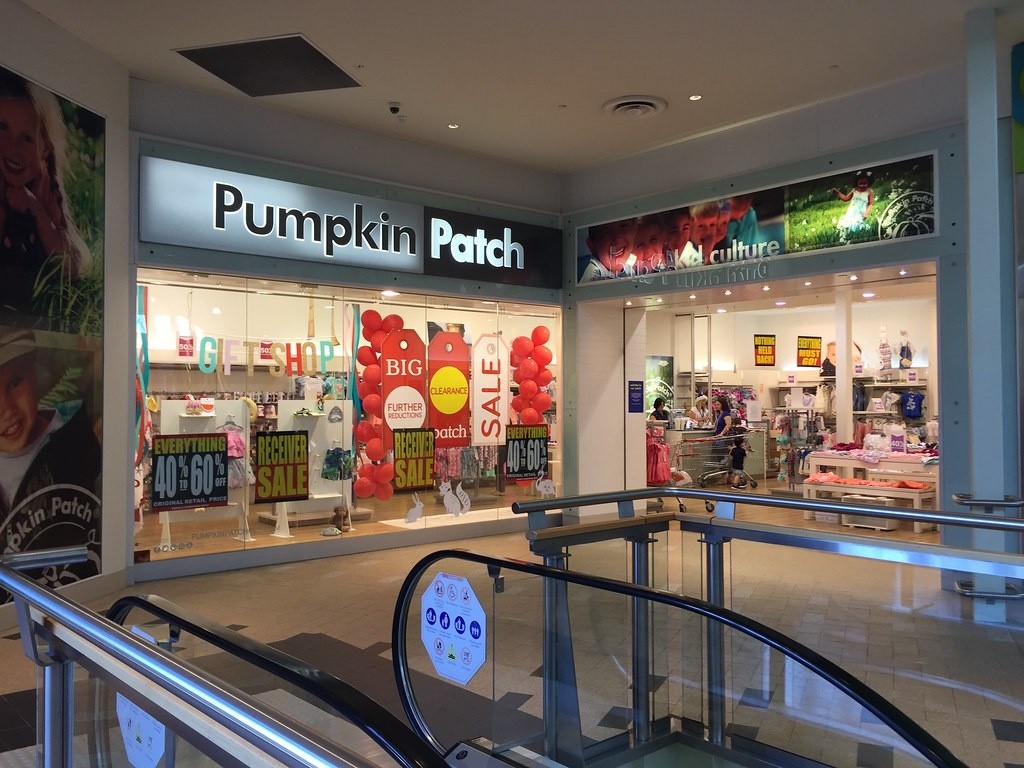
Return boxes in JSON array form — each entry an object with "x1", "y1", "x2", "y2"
[
  {"x1": 507, "y1": 326, "x2": 555, "y2": 437},
  {"x1": 349, "y1": 303, "x2": 424, "y2": 503}
]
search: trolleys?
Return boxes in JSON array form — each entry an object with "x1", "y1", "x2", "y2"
[
  {"x1": 663, "y1": 437, "x2": 715, "y2": 487},
  {"x1": 696, "y1": 430, "x2": 759, "y2": 488}
]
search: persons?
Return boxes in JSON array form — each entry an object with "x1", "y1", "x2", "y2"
[
  {"x1": 627, "y1": 208, "x2": 674, "y2": 277},
  {"x1": 893, "y1": 326, "x2": 916, "y2": 383},
  {"x1": 578, "y1": 214, "x2": 638, "y2": 286},
  {"x1": 657, "y1": 203, "x2": 704, "y2": 273},
  {"x1": 726, "y1": 194, "x2": 764, "y2": 268},
  {"x1": 0, "y1": 62, "x2": 105, "y2": 326},
  {"x1": 649, "y1": 396, "x2": 672, "y2": 430},
  {"x1": 712, "y1": 198, "x2": 734, "y2": 266},
  {"x1": 828, "y1": 168, "x2": 878, "y2": 237},
  {"x1": 686, "y1": 200, "x2": 721, "y2": 272},
  {"x1": 0, "y1": 323, "x2": 109, "y2": 602},
  {"x1": 822, "y1": 340, "x2": 841, "y2": 377},
  {"x1": 869, "y1": 324, "x2": 896, "y2": 386},
  {"x1": 729, "y1": 436, "x2": 750, "y2": 490},
  {"x1": 689, "y1": 390, "x2": 713, "y2": 430},
  {"x1": 709, "y1": 394, "x2": 735, "y2": 466}
]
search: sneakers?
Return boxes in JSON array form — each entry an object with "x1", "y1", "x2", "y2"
[
  {"x1": 739, "y1": 482, "x2": 747, "y2": 487},
  {"x1": 730, "y1": 484, "x2": 738, "y2": 489}
]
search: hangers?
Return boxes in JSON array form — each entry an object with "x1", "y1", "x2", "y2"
[
  {"x1": 908, "y1": 386, "x2": 915, "y2": 396},
  {"x1": 310, "y1": 375, "x2": 317, "y2": 379},
  {"x1": 334, "y1": 372, "x2": 342, "y2": 378},
  {"x1": 859, "y1": 417, "x2": 905, "y2": 437},
  {"x1": 928, "y1": 416, "x2": 938, "y2": 423},
  {"x1": 884, "y1": 387, "x2": 892, "y2": 397},
  {"x1": 852, "y1": 379, "x2": 859, "y2": 389},
  {"x1": 819, "y1": 381, "x2": 836, "y2": 390},
  {"x1": 803, "y1": 389, "x2": 810, "y2": 395}
]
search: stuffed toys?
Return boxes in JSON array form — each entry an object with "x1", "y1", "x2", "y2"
[{"x1": 329, "y1": 505, "x2": 351, "y2": 534}]
